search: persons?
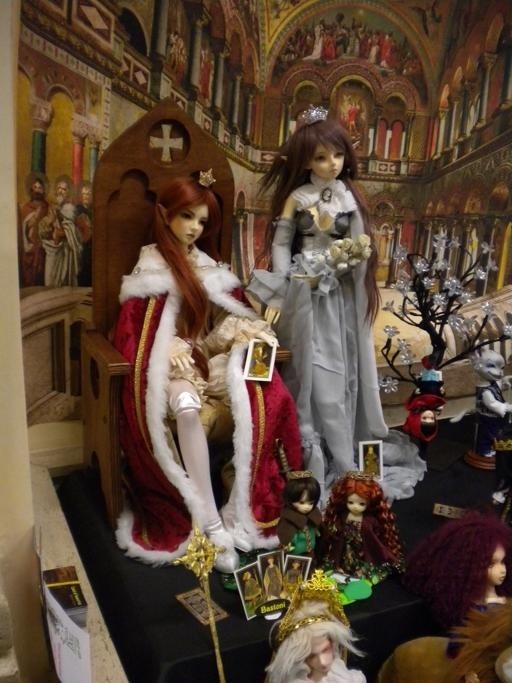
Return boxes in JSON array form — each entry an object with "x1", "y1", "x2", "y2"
[
  {"x1": 117, "y1": 171, "x2": 306, "y2": 573},
  {"x1": 271, "y1": 352, "x2": 511, "y2": 683},
  {"x1": 258, "y1": 108, "x2": 426, "y2": 504}
]
